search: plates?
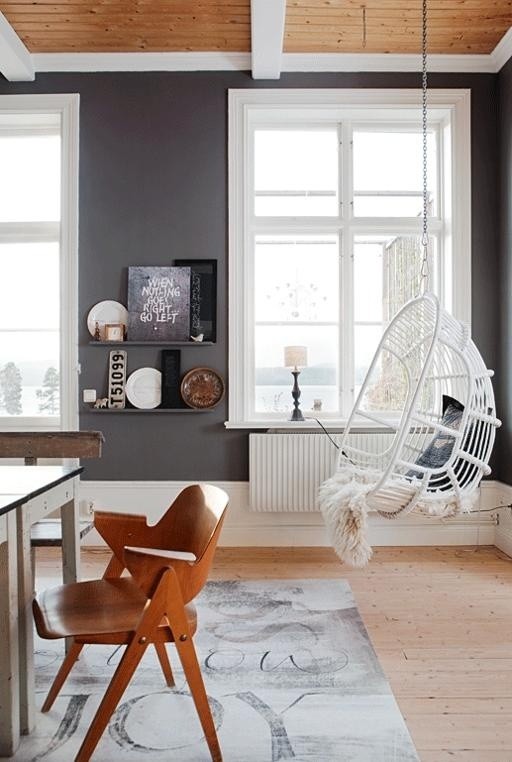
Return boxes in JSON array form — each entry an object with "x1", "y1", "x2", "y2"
[
  {"x1": 86, "y1": 299, "x2": 129, "y2": 341},
  {"x1": 180, "y1": 368, "x2": 225, "y2": 410},
  {"x1": 124, "y1": 366, "x2": 163, "y2": 409}
]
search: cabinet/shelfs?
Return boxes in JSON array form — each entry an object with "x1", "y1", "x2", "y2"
[{"x1": 86, "y1": 337, "x2": 216, "y2": 414}]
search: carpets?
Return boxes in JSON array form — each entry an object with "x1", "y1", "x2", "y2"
[{"x1": 2, "y1": 574, "x2": 415, "y2": 758}]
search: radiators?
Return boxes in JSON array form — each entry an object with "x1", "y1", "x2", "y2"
[{"x1": 249, "y1": 432, "x2": 431, "y2": 513}]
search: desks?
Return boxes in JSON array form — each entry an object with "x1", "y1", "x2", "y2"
[{"x1": 1, "y1": 463, "x2": 81, "y2": 760}]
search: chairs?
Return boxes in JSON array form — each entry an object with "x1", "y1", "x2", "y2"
[{"x1": 32, "y1": 481, "x2": 229, "y2": 762}]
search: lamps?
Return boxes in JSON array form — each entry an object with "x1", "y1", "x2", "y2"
[{"x1": 283, "y1": 345, "x2": 308, "y2": 422}]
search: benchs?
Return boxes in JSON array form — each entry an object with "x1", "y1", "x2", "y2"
[{"x1": 1, "y1": 430, "x2": 106, "y2": 553}]
